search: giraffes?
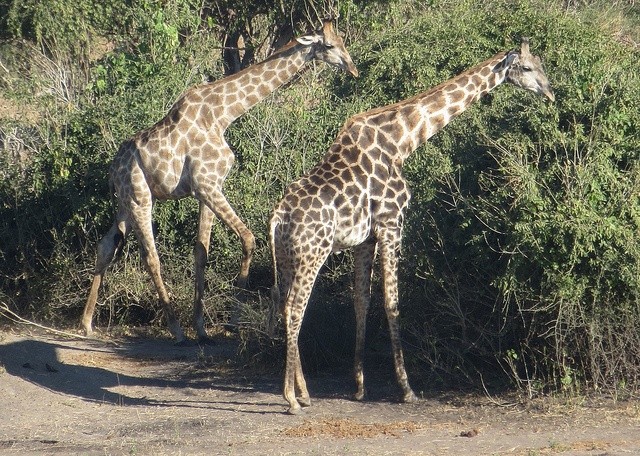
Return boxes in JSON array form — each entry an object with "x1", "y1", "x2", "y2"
[
  {"x1": 77, "y1": 17, "x2": 359, "y2": 356},
  {"x1": 266, "y1": 38, "x2": 557, "y2": 415}
]
